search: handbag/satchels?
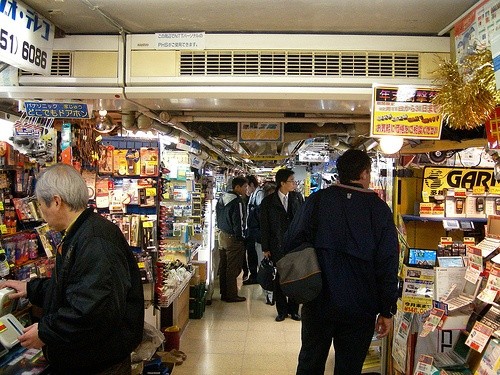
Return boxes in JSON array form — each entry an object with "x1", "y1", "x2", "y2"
[
  {"x1": 247, "y1": 203, "x2": 262, "y2": 244},
  {"x1": 276, "y1": 244, "x2": 323, "y2": 304},
  {"x1": 257, "y1": 257, "x2": 280, "y2": 306}
]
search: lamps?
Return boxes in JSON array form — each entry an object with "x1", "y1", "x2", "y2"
[
  {"x1": 361, "y1": 133, "x2": 379, "y2": 151},
  {"x1": 330, "y1": 134, "x2": 349, "y2": 146},
  {"x1": 94, "y1": 99, "x2": 117, "y2": 133}
]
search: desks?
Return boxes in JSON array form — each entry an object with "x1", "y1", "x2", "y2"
[{"x1": 158, "y1": 245, "x2": 208, "y2": 337}]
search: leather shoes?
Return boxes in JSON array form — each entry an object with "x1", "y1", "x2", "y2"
[
  {"x1": 221, "y1": 295, "x2": 246, "y2": 302},
  {"x1": 276, "y1": 314, "x2": 288, "y2": 321},
  {"x1": 289, "y1": 313, "x2": 300, "y2": 321}
]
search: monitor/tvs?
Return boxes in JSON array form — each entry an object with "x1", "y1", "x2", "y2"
[{"x1": 408, "y1": 248, "x2": 438, "y2": 268}]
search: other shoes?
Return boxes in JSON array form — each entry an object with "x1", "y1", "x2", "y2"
[
  {"x1": 243, "y1": 280, "x2": 258, "y2": 285},
  {"x1": 243, "y1": 273, "x2": 248, "y2": 279}
]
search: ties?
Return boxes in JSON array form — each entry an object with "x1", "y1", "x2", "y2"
[{"x1": 282, "y1": 196, "x2": 288, "y2": 212}]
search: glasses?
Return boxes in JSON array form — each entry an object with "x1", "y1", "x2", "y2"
[{"x1": 282, "y1": 179, "x2": 296, "y2": 183}]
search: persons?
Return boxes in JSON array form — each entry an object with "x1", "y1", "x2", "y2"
[
  {"x1": 279, "y1": 148, "x2": 397, "y2": 375},
  {"x1": 215, "y1": 169, "x2": 306, "y2": 321},
  {"x1": 0, "y1": 162, "x2": 145, "y2": 375}
]
